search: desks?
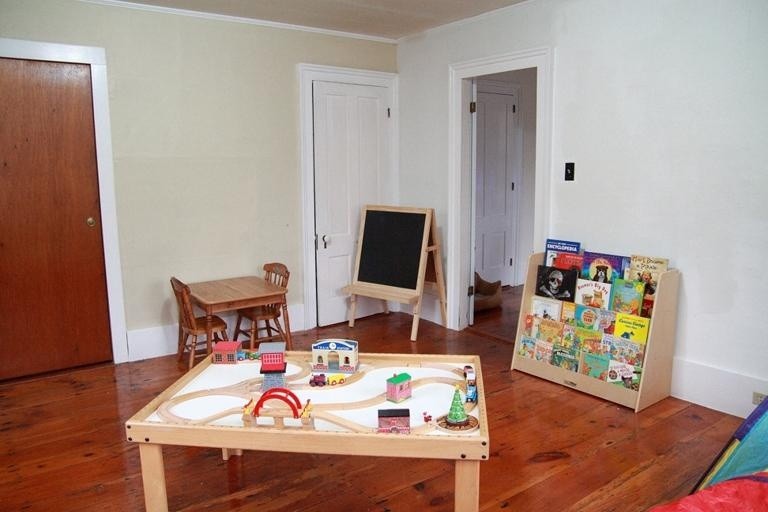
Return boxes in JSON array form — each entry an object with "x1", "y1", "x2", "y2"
[{"x1": 125, "y1": 350, "x2": 490, "y2": 512}]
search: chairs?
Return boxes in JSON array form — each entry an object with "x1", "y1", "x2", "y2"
[{"x1": 170, "y1": 263, "x2": 293, "y2": 371}]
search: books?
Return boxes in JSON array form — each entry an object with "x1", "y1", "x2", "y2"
[{"x1": 518, "y1": 237, "x2": 670, "y2": 392}]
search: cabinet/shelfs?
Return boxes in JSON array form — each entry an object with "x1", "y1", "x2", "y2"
[{"x1": 510, "y1": 253, "x2": 680, "y2": 413}]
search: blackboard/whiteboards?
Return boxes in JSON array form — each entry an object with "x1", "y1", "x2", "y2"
[{"x1": 354, "y1": 205, "x2": 432, "y2": 294}]
